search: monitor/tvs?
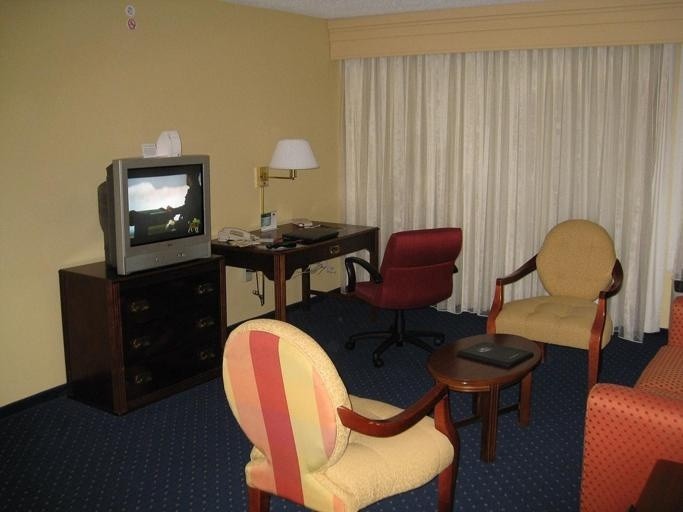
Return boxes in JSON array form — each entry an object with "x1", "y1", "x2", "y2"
[{"x1": 97, "y1": 155, "x2": 212, "y2": 277}]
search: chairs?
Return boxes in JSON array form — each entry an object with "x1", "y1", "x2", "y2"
[
  {"x1": 220, "y1": 318, "x2": 460, "y2": 512},
  {"x1": 343, "y1": 228, "x2": 462, "y2": 368},
  {"x1": 580, "y1": 298, "x2": 683, "y2": 512},
  {"x1": 487, "y1": 219, "x2": 624, "y2": 392}
]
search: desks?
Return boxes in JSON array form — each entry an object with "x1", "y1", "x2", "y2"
[{"x1": 211, "y1": 218, "x2": 379, "y2": 349}]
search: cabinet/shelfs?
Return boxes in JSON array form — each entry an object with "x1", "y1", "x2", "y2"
[{"x1": 59, "y1": 255, "x2": 227, "y2": 416}]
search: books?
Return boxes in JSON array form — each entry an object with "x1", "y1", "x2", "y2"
[
  {"x1": 457, "y1": 343, "x2": 534, "y2": 368},
  {"x1": 282, "y1": 228, "x2": 339, "y2": 245}
]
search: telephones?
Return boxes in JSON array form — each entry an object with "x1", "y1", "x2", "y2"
[{"x1": 218, "y1": 227, "x2": 256, "y2": 242}]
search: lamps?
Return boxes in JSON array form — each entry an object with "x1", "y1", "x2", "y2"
[{"x1": 252, "y1": 139, "x2": 321, "y2": 225}]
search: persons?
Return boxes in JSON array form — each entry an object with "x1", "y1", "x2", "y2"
[{"x1": 167, "y1": 172, "x2": 203, "y2": 234}]
